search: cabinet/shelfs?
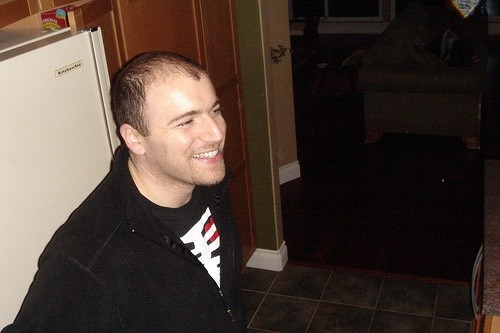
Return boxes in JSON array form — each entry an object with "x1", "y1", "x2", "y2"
[
  {"x1": 118, "y1": 0, "x2": 257, "y2": 273},
  {"x1": 0, "y1": 0, "x2": 128, "y2": 83},
  {"x1": 470, "y1": 262, "x2": 500, "y2": 333}
]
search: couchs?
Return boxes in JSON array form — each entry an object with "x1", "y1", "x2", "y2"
[{"x1": 357, "y1": 0, "x2": 492, "y2": 148}]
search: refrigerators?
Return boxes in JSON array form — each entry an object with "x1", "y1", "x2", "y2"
[{"x1": 0, "y1": 26, "x2": 125, "y2": 333}]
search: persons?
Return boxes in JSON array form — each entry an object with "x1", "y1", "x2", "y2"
[{"x1": 0, "y1": 51, "x2": 246, "y2": 333}]
